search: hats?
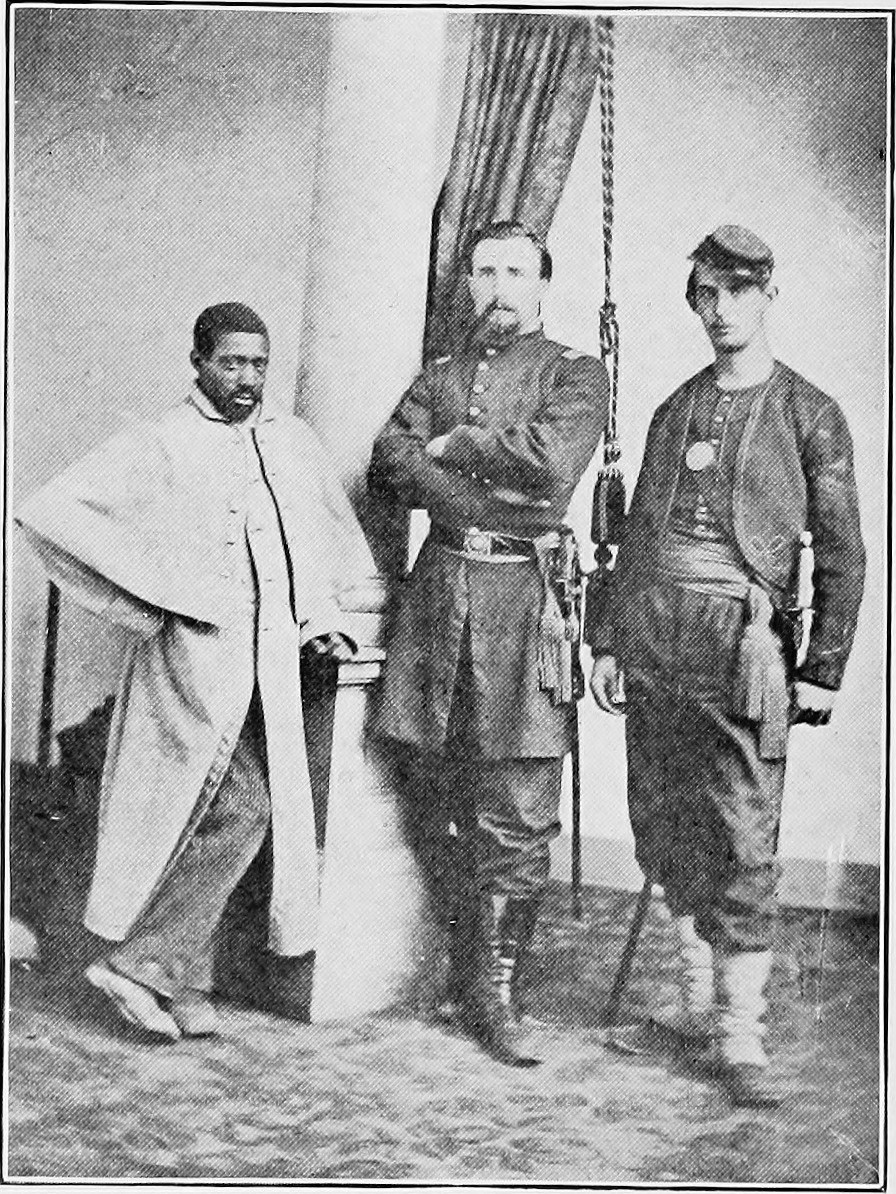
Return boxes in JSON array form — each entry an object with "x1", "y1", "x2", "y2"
[{"x1": 706, "y1": 225, "x2": 774, "y2": 279}]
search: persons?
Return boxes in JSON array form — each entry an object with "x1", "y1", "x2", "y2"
[
  {"x1": 8, "y1": 303, "x2": 384, "y2": 1040},
  {"x1": 366, "y1": 218, "x2": 611, "y2": 1061},
  {"x1": 591, "y1": 225, "x2": 867, "y2": 1107}
]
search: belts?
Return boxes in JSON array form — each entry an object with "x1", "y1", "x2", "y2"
[{"x1": 431, "y1": 524, "x2": 549, "y2": 559}]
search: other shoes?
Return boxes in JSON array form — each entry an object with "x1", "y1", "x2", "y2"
[
  {"x1": 170, "y1": 986, "x2": 223, "y2": 1034},
  {"x1": 83, "y1": 955, "x2": 183, "y2": 1041}
]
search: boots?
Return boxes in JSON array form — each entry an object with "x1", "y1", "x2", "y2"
[
  {"x1": 650, "y1": 916, "x2": 715, "y2": 1043},
  {"x1": 712, "y1": 949, "x2": 782, "y2": 1104},
  {"x1": 438, "y1": 889, "x2": 548, "y2": 1065}
]
